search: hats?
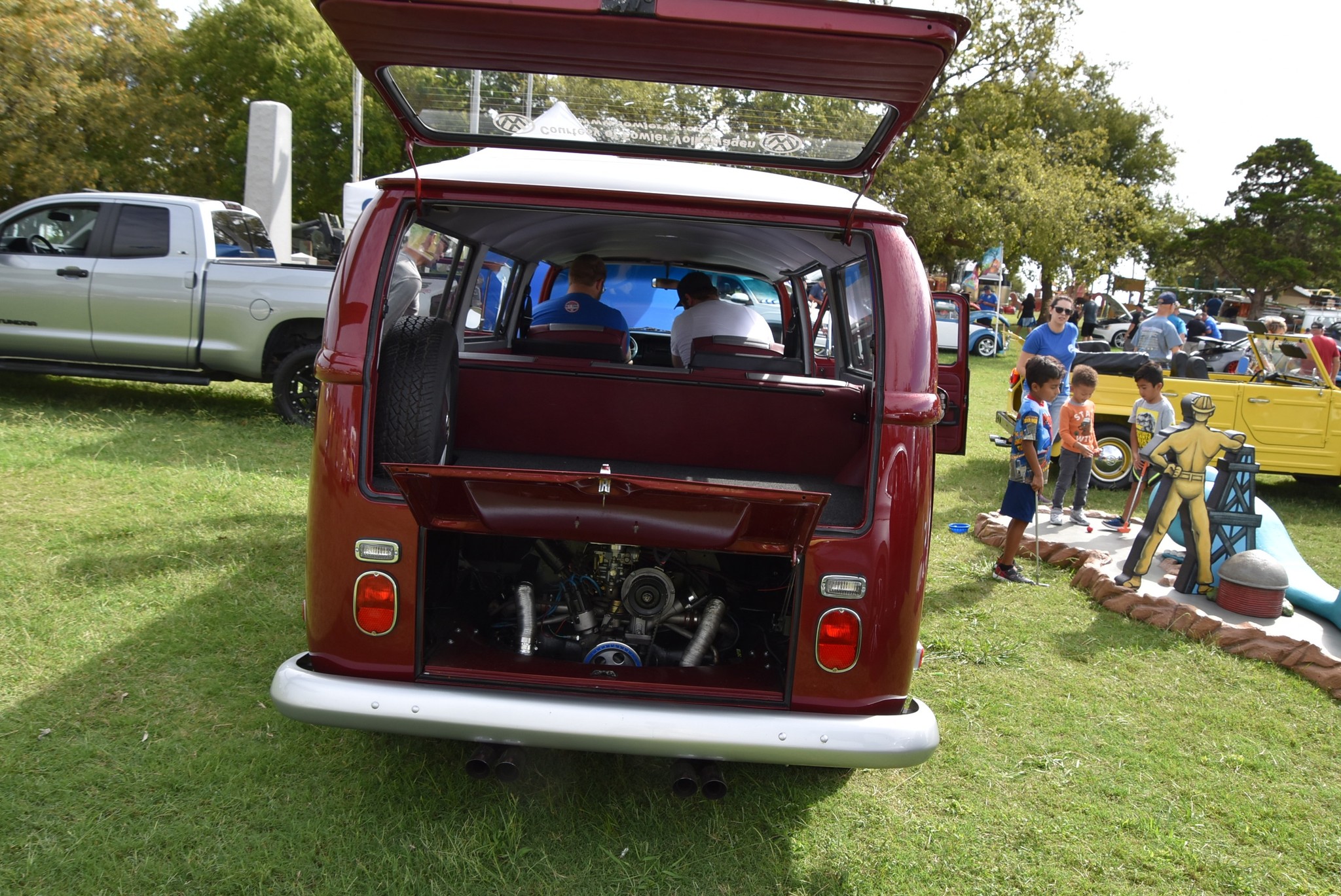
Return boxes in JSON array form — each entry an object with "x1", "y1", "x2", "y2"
[
  {"x1": 984, "y1": 286, "x2": 991, "y2": 290},
  {"x1": 1311, "y1": 321, "x2": 1325, "y2": 329},
  {"x1": 1136, "y1": 303, "x2": 1145, "y2": 308},
  {"x1": 432, "y1": 230, "x2": 459, "y2": 245},
  {"x1": 673, "y1": 272, "x2": 713, "y2": 310},
  {"x1": 1157, "y1": 291, "x2": 1177, "y2": 304}
]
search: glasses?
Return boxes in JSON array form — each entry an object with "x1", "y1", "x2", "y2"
[
  {"x1": 601, "y1": 284, "x2": 606, "y2": 293},
  {"x1": 429, "y1": 233, "x2": 450, "y2": 253},
  {"x1": 1053, "y1": 307, "x2": 1073, "y2": 315}
]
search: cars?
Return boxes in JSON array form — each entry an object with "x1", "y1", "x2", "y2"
[
  {"x1": 989, "y1": 330, "x2": 1341, "y2": 490},
  {"x1": 934, "y1": 298, "x2": 1011, "y2": 357},
  {"x1": 1092, "y1": 288, "x2": 1341, "y2": 388}
]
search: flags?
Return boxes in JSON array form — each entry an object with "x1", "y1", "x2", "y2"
[{"x1": 961, "y1": 246, "x2": 1001, "y2": 291}]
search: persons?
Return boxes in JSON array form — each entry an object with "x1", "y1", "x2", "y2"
[
  {"x1": 1130, "y1": 291, "x2": 1184, "y2": 369},
  {"x1": 1241, "y1": 315, "x2": 1287, "y2": 375},
  {"x1": 530, "y1": 254, "x2": 634, "y2": 365},
  {"x1": 1185, "y1": 313, "x2": 1207, "y2": 355},
  {"x1": 1014, "y1": 293, "x2": 1037, "y2": 336},
  {"x1": 381, "y1": 227, "x2": 452, "y2": 341},
  {"x1": 1204, "y1": 294, "x2": 1222, "y2": 316},
  {"x1": 1050, "y1": 364, "x2": 1103, "y2": 526},
  {"x1": 1201, "y1": 312, "x2": 1222, "y2": 340},
  {"x1": 808, "y1": 278, "x2": 828, "y2": 310},
  {"x1": 1101, "y1": 361, "x2": 1175, "y2": 529},
  {"x1": 1067, "y1": 303, "x2": 1080, "y2": 326},
  {"x1": 1016, "y1": 293, "x2": 1079, "y2": 505},
  {"x1": 790, "y1": 278, "x2": 807, "y2": 317},
  {"x1": 1304, "y1": 328, "x2": 1310, "y2": 334},
  {"x1": 670, "y1": 271, "x2": 775, "y2": 368},
  {"x1": 993, "y1": 354, "x2": 1069, "y2": 585},
  {"x1": 1123, "y1": 303, "x2": 1148, "y2": 352},
  {"x1": 976, "y1": 285, "x2": 997, "y2": 327},
  {"x1": 1167, "y1": 301, "x2": 1188, "y2": 369},
  {"x1": 1077, "y1": 292, "x2": 1098, "y2": 341},
  {"x1": 1292, "y1": 322, "x2": 1340, "y2": 385}
]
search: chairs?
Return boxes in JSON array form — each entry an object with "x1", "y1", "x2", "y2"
[
  {"x1": 1187, "y1": 356, "x2": 1209, "y2": 379},
  {"x1": 1170, "y1": 350, "x2": 1192, "y2": 378},
  {"x1": 526, "y1": 322, "x2": 626, "y2": 364},
  {"x1": 689, "y1": 335, "x2": 803, "y2": 373},
  {"x1": 719, "y1": 282, "x2": 732, "y2": 300}
]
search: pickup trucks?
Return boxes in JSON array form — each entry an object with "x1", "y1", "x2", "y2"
[{"x1": 0, "y1": 195, "x2": 338, "y2": 430}]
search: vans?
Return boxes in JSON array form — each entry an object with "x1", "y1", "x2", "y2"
[{"x1": 269, "y1": 0, "x2": 971, "y2": 767}]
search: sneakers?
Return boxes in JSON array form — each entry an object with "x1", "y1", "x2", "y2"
[
  {"x1": 993, "y1": 560, "x2": 1035, "y2": 585},
  {"x1": 1069, "y1": 509, "x2": 1090, "y2": 525},
  {"x1": 1050, "y1": 507, "x2": 1064, "y2": 525},
  {"x1": 1101, "y1": 515, "x2": 1131, "y2": 530},
  {"x1": 994, "y1": 553, "x2": 1024, "y2": 572}
]
